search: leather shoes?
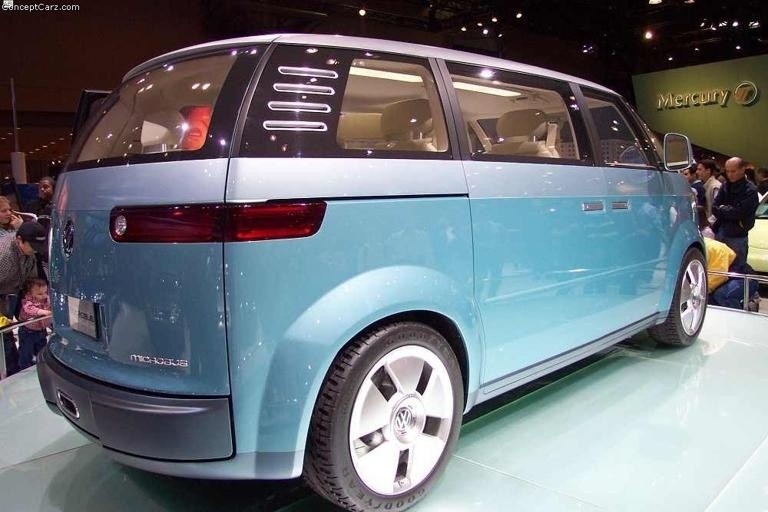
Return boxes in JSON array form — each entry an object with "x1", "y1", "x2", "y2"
[{"x1": 742, "y1": 299, "x2": 758, "y2": 312}]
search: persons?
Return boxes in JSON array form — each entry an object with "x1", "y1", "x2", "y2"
[
  {"x1": 462, "y1": 201, "x2": 680, "y2": 295},
  {"x1": 683, "y1": 156, "x2": 768, "y2": 310},
  {"x1": 0, "y1": 177, "x2": 55, "y2": 377}
]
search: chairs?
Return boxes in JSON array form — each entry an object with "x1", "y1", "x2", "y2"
[
  {"x1": 138, "y1": 107, "x2": 186, "y2": 154},
  {"x1": 481, "y1": 105, "x2": 556, "y2": 163},
  {"x1": 372, "y1": 97, "x2": 442, "y2": 155}
]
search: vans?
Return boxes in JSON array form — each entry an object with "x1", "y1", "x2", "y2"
[{"x1": 30, "y1": 31, "x2": 712, "y2": 511}]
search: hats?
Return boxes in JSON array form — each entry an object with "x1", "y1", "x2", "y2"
[{"x1": 19, "y1": 223, "x2": 49, "y2": 254}]
